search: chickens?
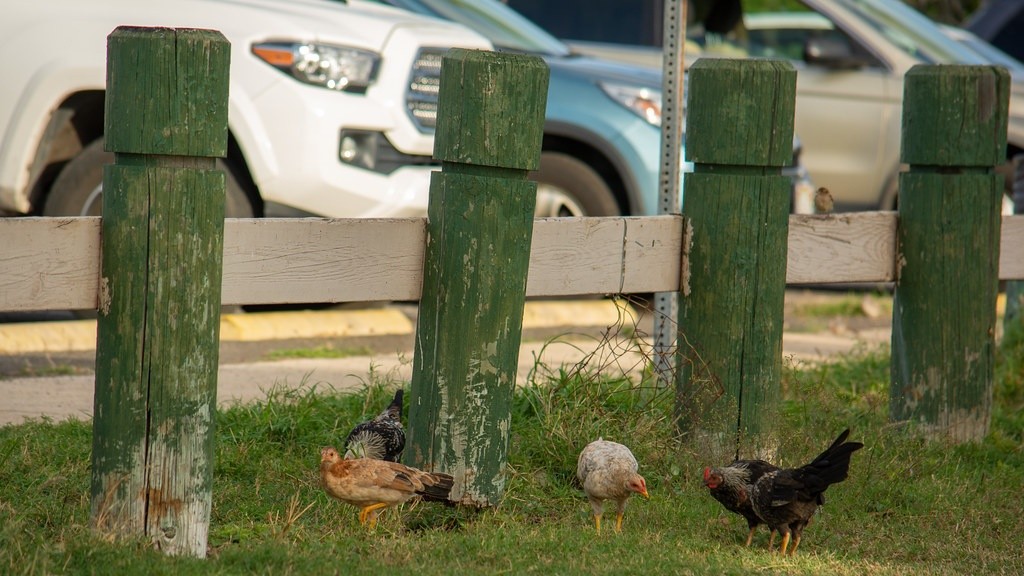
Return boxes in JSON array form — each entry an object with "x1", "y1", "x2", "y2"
[
  {"x1": 703, "y1": 429, "x2": 865, "y2": 556},
  {"x1": 343, "y1": 389, "x2": 406, "y2": 465},
  {"x1": 577, "y1": 437, "x2": 649, "y2": 535},
  {"x1": 320, "y1": 446, "x2": 455, "y2": 529}
]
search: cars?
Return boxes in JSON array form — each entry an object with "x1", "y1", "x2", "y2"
[
  {"x1": 384, "y1": 1, "x2": 687, "y2": 218},
  {"x1": 685, "y1": 0, "x2": 1024, "y2": 211}
]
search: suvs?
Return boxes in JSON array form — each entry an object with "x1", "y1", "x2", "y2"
[{"x1": 0, "y1": 1, "x2": 500, "y2": 314}]
